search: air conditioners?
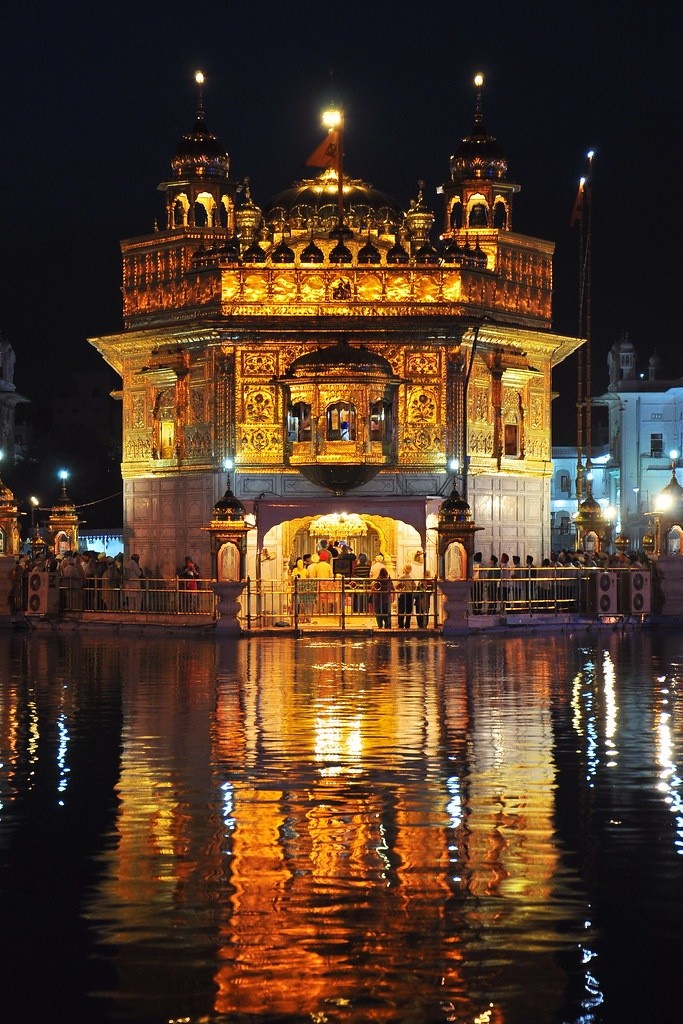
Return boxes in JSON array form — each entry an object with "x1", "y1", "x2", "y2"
[
  {"x1": 589, "y1": 572, "x2": 617, "y2": 614},
  {"x1": 28, "y1": 572, "x2": 60, "y2": 614},
  {"x1": 620, "y1": 572, "x2": 651, "y2": 612}
]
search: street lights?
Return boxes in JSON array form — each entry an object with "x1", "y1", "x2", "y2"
[
  {"x1": 29, "y1": 497, "x2": 39, "y2": 536},
  {"x1": 575, "y1": 148, "x2": 597, "y2": 510}
]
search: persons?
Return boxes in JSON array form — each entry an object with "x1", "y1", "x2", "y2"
[
  {"x1": 1, "y1": 549, "x2": 146, "y2": 615},
  {"x1": 282, "y1": 540, "x2": 664, "y2": 629},
  {"x1": 179, "y1": 556, "x2": 202, "y2": 614}
]
